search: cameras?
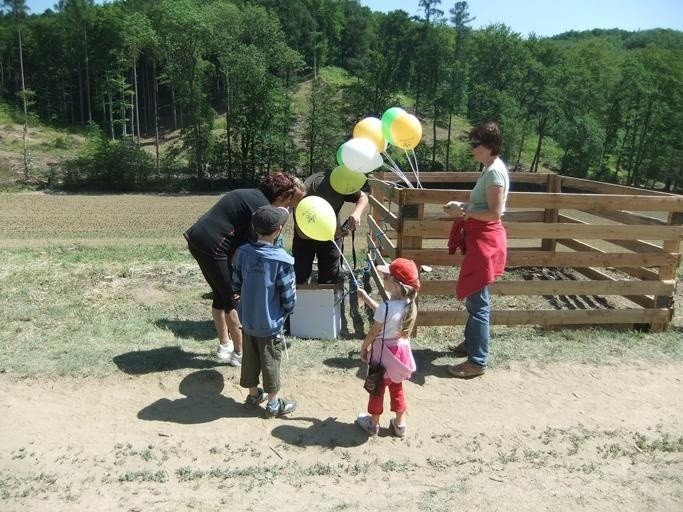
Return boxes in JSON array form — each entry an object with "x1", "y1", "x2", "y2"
[{"x1": 342, "y1": 218, "x2": 357, "y2": 234}]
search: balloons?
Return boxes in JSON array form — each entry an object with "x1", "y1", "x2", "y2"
[
  {"x1": 296, "y1": 195, "x2": 338, "y2": 242},
  {"x1": 391, "y1": 114, "x2": 423, "y2": 150},
  {"x1": 352, "y1": 116, "x2": 387, "y2": 154},
  {"x1": 341, "y1": 137, "x2": 383, "y2": 173},
  {"x1": 381, "y1": 107, "x2": 403, "y2": 145},
  {"x1": 336, "y1": 143, "x2": 345, "y2": 166},
  {"x1": 330, "y1": 166, "x2": 367, "y2": 195}
]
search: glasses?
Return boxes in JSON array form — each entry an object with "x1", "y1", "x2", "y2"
[{"x1": 468, "y1": 142, "x2": 483, "y2": 149}]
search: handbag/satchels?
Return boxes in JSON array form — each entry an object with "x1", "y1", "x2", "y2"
[{"x1": 363, "y1": 364, "x2": 386, "y2": 397}]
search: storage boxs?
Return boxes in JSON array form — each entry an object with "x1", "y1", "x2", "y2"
[{"x1": 288, "y1": 279, "x2": 343, "y2": 339}]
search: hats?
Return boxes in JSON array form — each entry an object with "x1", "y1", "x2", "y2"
[
  {"x1": 376, "y1": 257, "x2": 421, "y2": 290},
  {"x1": 252, "y1": 206, "x2": 289, "y2": 237}
]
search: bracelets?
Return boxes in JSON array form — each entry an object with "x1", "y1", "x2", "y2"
[{"x1": 460, "y1": 205, "x2": 467, "y2": 216}]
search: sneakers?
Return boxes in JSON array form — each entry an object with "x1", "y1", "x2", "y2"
[
  {"x1": 390, "y1": 416, "x2": 407, "y2": 437},
  {"x1": 265, "y1": 398, "x2": 297, "y2": 419},
  {"x1": 448, "y1": 342, "x2": 468, "y2": 355},
  {"x1": 216, "y1": 339, "x2": 234, "y2": 364},
  {"x1": 230, "y1": 351, "x2": 243, "y2": 367},
  {"x1": 356, "y1": 413, "x2": 380, "y2": 437},
  {"x1": 245, "y1": 388, "x2": 268, "y2": 410},
  {"x1": 447, "y1": 359, "x2": 485, "y2": 378}
]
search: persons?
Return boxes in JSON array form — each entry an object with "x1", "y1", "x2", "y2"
[
  {"x1": 356, "y1": 257, "x2": 421, "y2": 438},
  {"x1": 183, "y1": 172, "x2": 295, "y2": 368},
  {"x1": 231, "y1": 205, "x2": 297, "y2": 418},
  {"x1": 292, "y1": 167, "x2": 369, "y2": 285},
  {"x1": 444, "y1": 120, "x2": 510, "y2": 378},
  {"x1": 288, "y1": 176, "x2": 305, "y2": 208}
]
